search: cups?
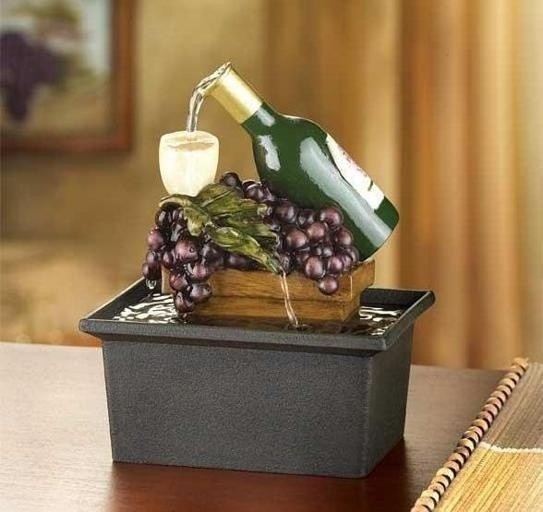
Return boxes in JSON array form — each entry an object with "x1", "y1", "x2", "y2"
[{"x1": 156, "y1": 130, "x2": 220, "y2": 194}]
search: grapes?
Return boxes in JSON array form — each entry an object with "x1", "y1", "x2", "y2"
[{"x1": 141, "y1": 173, "x2": 359, "y2": 311}]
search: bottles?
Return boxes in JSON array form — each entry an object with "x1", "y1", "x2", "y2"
[{"x1": 200, "y1": 62, "x2": 399, "y2": 260}]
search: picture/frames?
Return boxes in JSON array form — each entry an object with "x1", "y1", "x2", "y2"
[{"x1": 1, "y1": 0, "x2": 137, "y2": 156}]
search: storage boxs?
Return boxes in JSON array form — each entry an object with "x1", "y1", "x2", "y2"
[{"x1": 83, "y1": 269, "x2": 434, "y2": 481}]
